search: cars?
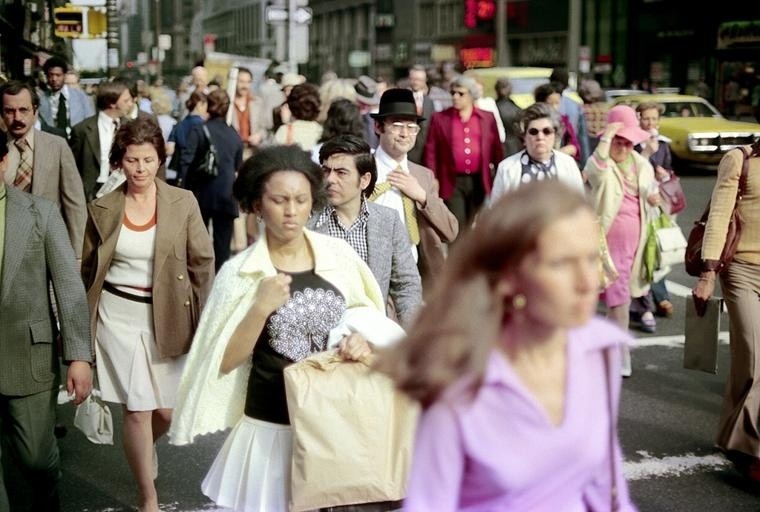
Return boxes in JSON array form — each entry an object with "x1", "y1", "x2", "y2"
[
  {"x1": 608, "y1": 92, "x2": 760, "y2": 168},
  {"x1": 452, "y1": 63, "x2": 587, "y2": 122},
  {"x1": 603, "y1": 87, "x2": 651, "y2": 106}
]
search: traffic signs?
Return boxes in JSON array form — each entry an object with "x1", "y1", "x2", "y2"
[
  {"x1": 264, "y1": 3, "x2": 315, "y2": 26},
  {"x1": 346, "y1": 50, "x2": 372, "y2": 68},
  {"x1": 374, "y1": 43, "x2": 396, "y2": 63},
  {"x1": 374, "y1": 12, "x2": 397, "y2": 29}
]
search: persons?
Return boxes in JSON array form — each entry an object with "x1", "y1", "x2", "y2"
[
  {"x1": 680, "y1": 105, "x2": 691, "y2": 116},
  {"x1": 691, "y1": 136, "x2": 760, "y2": 485}
]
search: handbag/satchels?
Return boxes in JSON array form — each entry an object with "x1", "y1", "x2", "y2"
[
  {"x1": 655, "y1": 168, "x2": 687, "y2": 214},
  {"x1": 283, "y1": 345, "x2": 423, "y2": 512},
  {"x1": 189, "y1": 148, "x2": 222, "y2": 180},
  {"x1": 653, "y1": 226, "x2": 689, "y2": 267},
  {"x1": 72, "y1": 396, "x2": 115, "y2": 444},
  {"x1": 682, "y1": 146, "x2": 749, "y2": 278}
]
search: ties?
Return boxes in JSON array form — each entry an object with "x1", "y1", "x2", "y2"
[
  {"x1": 108, "y1": 120, "x2": 120, "y2": 177},
  {"x1": 14, "y1": 139, "x2": 34, "y2": 196},
  {"x1": 369, "y1": 164, "x2": 422, "y2": 245},
  {"x1": 56, "y1": 92, "x2": 68, "y2": 130}
]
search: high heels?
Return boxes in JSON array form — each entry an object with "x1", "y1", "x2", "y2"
[{"x1": 641, "y1": 301, "x2": 673, "y2": 333}]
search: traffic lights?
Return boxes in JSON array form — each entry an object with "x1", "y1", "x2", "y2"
[
  {"x1": 53, "y1": 6, "x2": 83, "y2": 38},
  {"x1": 87, "y1": 9, "x2": 106, "y2": 35}
]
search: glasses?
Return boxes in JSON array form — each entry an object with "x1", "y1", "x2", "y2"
[
  {"x1": 528, "y1": 127, "x2": 556, "y2": 135},
  {"x1": 384, "y1": 119, "x2": 421, "y2": 136},
  {"x1": 449, "y1": 89, "x2": 470, "y2": 97}
]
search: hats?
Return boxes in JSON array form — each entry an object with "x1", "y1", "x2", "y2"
[
  {"x1": 595, "y1": 104, "x2": 650, "y2": 146},
  {"x1": 369, "y1": 87, "x2": 427, "y2": 123},
  {"x1": 350, "y1": 74, "x2": 381, "y2": 106}
]
